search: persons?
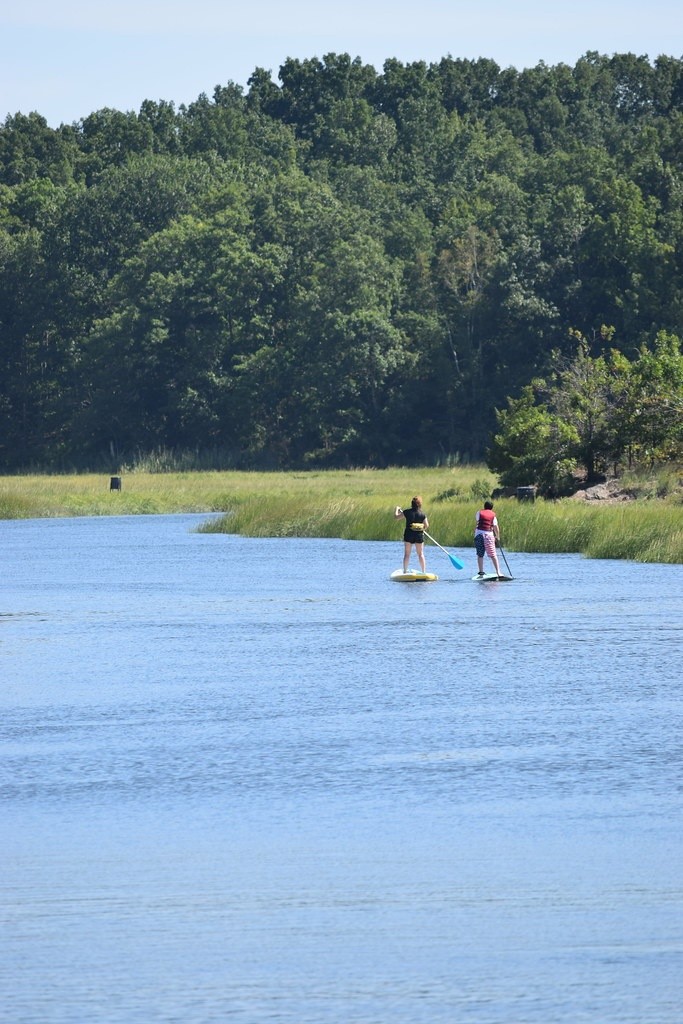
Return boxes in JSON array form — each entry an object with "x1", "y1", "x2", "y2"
[
  {"x1": 394, "y1": 496, "x2": 429, "y2": 574},
  {"x1": 474, "y1": 501, "x2": 504, "y2": 577}
]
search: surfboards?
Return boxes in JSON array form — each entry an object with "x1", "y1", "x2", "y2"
[
  {"x1": 471, "y1": 572, "x2": 513, "y2": 583},
  {"x1": 390, "y1": 569, "x2": 438, "y2": 582}
]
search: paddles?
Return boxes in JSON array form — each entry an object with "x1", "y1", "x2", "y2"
[
  {"x1": 398, "y1": 508, "x2": 465, "y2": 570},
  {"x1": 492, "y1": 528, "x2": 515, "y2": 578}
]
